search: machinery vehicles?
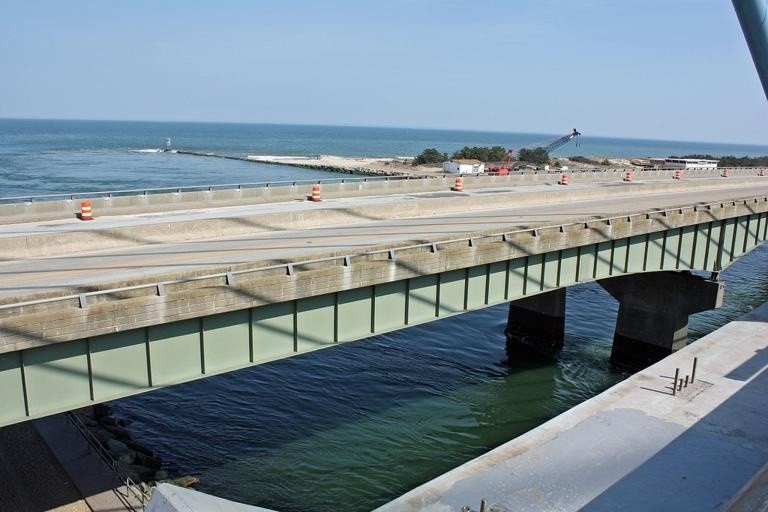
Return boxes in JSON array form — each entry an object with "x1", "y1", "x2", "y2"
[{"x1": 486, "y1": 128, "x2": 581, "y2": 175}]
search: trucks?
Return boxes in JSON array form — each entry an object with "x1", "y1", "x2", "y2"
[{"x1": 558, "y1": 166, "x2": 567, "y2": 170}]
